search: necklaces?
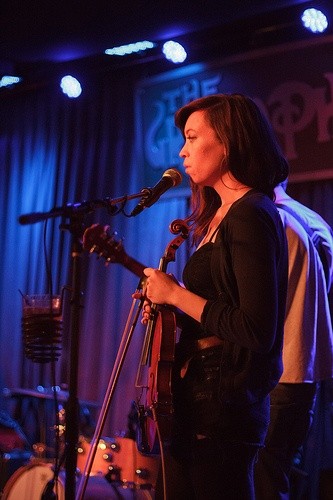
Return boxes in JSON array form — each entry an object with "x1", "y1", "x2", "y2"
[{"x1": 201, "y1": 184, "x2": 247, "y2": 244}]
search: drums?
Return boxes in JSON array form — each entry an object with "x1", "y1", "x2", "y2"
[
  {"x1": 76, "y1": 437, "x2": 156, "y2": 497},
  {"x1": 3, "y1": 460, "x2": 117, "y2": 500}
]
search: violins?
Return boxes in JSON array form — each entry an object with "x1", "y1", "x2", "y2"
[{"x1": 145, "y1": 219, "x2": 190, "y2": 455}]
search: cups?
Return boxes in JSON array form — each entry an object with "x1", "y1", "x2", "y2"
[{"x1": 23, "y1": 295, "x2": 62, "y2": 346}]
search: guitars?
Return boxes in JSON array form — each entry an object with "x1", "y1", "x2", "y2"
[{"x1": 80, "y1": 222, "x2": 146, "y2": 279}]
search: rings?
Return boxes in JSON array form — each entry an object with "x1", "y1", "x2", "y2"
[{"x1": 145, "y1": 281, "x2": 149, "y2": 286}]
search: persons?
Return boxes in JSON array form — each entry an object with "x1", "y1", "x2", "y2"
[
  {"x1": 141, "y1": 94, "x2": 288, "y2": 500},
  {"x1": 254, "y1": 183, "x2": 333, "y2": 500}
]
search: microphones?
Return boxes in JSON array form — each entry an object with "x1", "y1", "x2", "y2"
[{"x1": 130, "y1": 168, "x2": 183, "y2": 216}]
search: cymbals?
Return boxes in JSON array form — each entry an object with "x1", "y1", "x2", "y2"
[{"x1": 15, "y1": 387, "x2": 101, "y2": 409}]
